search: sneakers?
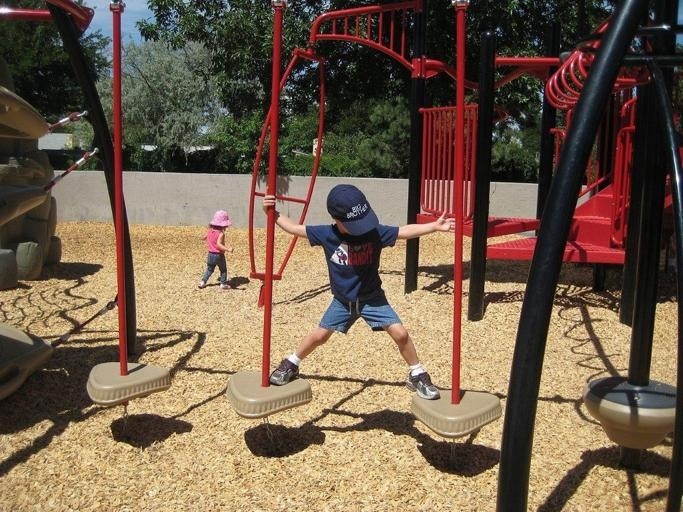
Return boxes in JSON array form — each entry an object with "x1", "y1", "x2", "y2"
[
  {"x1": 199, "y1": 281, "x2": 206, "y2": 287},
  {"x1": 220, "y1": 284, "x2": 230, "y2": 289},
  {"x1": 272, "y1": 358, "x2": 299, "y2": 385},
  {"x1": 405, "y1": 371, "x2": 439, "y2": 399}
]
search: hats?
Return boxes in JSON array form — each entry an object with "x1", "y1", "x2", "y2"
[
  {"x1": 327, "y1": 185, "x2": 380, "y2": 236},
  {"x1": 209, "y1": 209, "x2": 232, "y2": 228}
]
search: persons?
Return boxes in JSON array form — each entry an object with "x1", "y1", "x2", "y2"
[
  {"x1": 194, "y1": 210, "x2": 235, "y2": 290},
  {"x1": 260, "y1": 182, "x2": 455, "y2": 401}
]
